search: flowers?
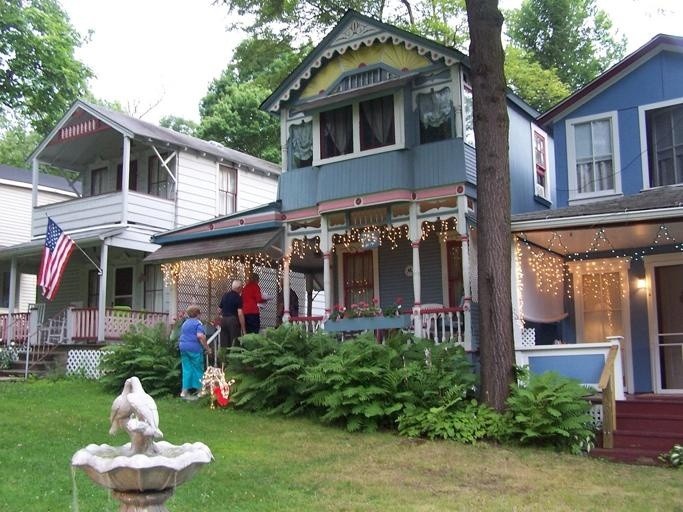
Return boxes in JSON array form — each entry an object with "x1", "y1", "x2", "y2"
[{"x1": 333, "y1": 296, "x2": 406, "y2": 322}]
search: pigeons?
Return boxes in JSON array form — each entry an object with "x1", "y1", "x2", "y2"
[
  {"x1": 107, "y1": 377, "x2": 131, "y2": 438},
  {"x1": 127, "y1": 377, "x2": 163, "y2": 440}
]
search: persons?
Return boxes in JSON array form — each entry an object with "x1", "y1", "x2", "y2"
[
  {"x1": 273, "y1": 277, "x2": 301, "y2": 329},
  {"x1": 177, "y1": 304, "x2": 211, "y2": 399},
  {"x1": 215, "y1": 278, "x2": 247, "y2": 364},
  {"x1": 240, "y1": 272, "x2": 274, "y2": 336}
]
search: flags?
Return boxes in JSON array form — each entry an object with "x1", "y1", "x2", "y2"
[{"x1": 35, "y1": 216, "x2": 76, "y2": 303}]
